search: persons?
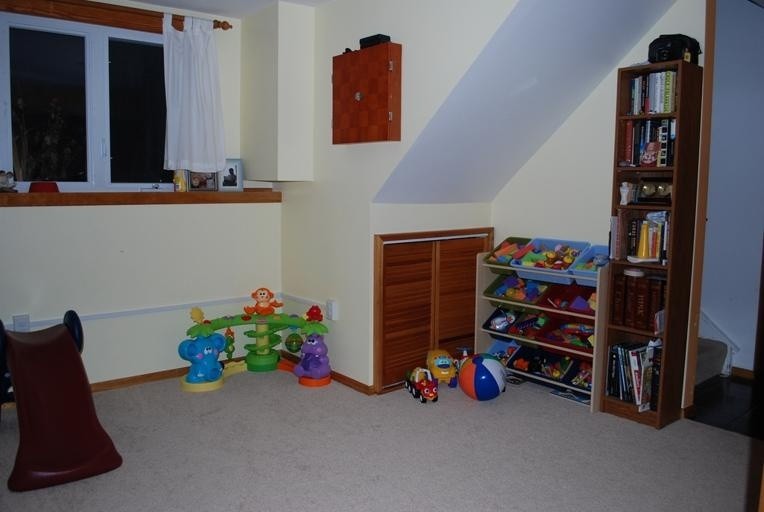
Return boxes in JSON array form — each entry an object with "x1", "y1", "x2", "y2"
[{"x1": 223, "y1": 168, "x2": 236, "y2": 186}]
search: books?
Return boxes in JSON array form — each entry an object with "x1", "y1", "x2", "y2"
[{"x1": 606, "y1": 70, "x2": 678, "y2": 415}]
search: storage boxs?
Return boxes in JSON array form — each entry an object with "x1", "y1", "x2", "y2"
[{"x1": 480, "y1": 234, "x2": 609, "y2": 397}]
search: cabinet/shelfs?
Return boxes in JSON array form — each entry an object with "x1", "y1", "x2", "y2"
[
  {"x1": 327, "y1": 41, "x2": 403, "y2": 145},
  {"x1": 468, "y1": 251, "x2": 602, "y2": 414},
  {"x1": 597, "y1": 60, "x2": 704, "y2": 431}
]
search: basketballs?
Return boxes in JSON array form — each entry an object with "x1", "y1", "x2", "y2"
[{"x1": 457, "y1": 354, "x2": 506, "y2": 401}]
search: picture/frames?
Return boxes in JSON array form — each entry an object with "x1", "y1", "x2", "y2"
[
  {"x1": 184, "y1": 170, "x2": 219, "y2": 192},
  {"x1": 218, "y1": 158, "x2": 244, "y2": 192}
]
